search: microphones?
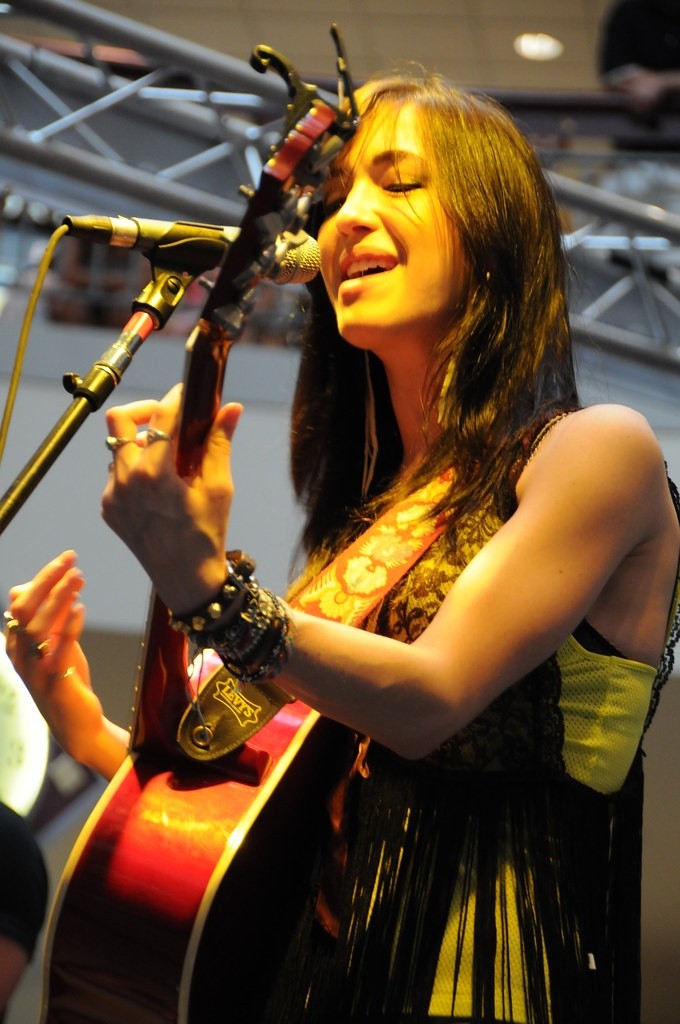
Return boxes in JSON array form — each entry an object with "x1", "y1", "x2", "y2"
[{"x1": 63, "y1": 211, "x2": 324, "y2": 288}]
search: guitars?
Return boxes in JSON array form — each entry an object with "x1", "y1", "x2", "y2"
[{"x1": 19, "y1": 24, "x2": 362, "y2": 1024}]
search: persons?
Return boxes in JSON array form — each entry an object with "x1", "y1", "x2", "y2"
[
  {"x1": 4, "y1": 74, "x2": 680, "y2": 1024},
  {"x1": 0, "y1": 802, "x2": 49, "y2": 1024}
]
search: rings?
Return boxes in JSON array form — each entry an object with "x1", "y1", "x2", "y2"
[
  {"x1": 31, "y1": 644, "x2": 49, "y2": 656},
  {"x1": 108, "y1": 463, "x2": 115, "y2": 472},
  {"x1": 105, "y1": 436, "x2": 134, "y2": 450},
  {"x1": 147, "y1": 428, "x2": 172, "y2": 443},
  {"x1": 7, "y1": 619, "x2": 25, "y2": 633},
  {"x1": 4, "y1": 611, "x2": 13, "y2": 621}
]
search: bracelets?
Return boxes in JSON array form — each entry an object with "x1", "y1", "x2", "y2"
[{"x1": 166, "y1": 548, "x2": 295, "y2": 683}]
